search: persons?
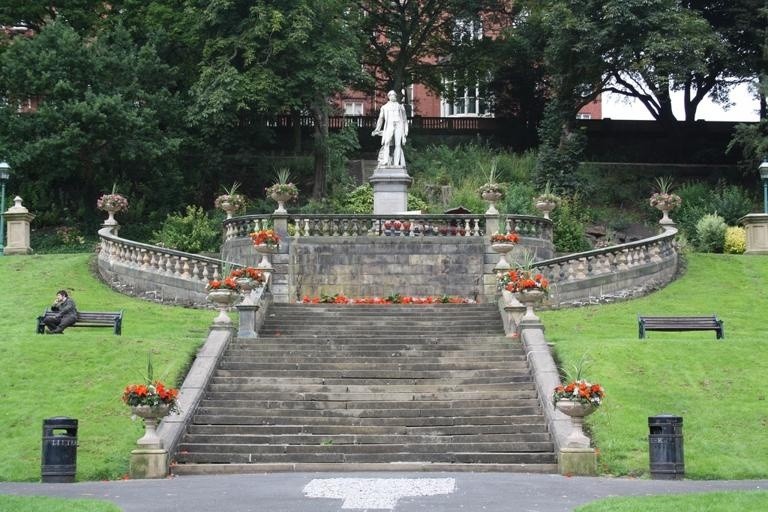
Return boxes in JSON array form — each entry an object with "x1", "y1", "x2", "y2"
[
  {"x1": 371, "y1": 90, "x2": 409, "y2": 167},
  {"x1": 42, "y1": 289, "x2": 79, "y2": 335}
]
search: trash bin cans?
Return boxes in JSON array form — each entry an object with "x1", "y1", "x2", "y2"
[
  {"x1": 648, "y1": 413, "x2": 685, "y2": 481},
  {"x1": 41, "y1": 416, "x2": 78, "y2": 482}
]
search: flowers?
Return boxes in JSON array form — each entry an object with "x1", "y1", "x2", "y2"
[
  {"x1": 495, "y1": 246, "x2": 550, "y2": 301},
  {"x1": 204, "y1": 247, "x2": 239, "y2": 294},
  {"x1": 120, "y1": 351, "x2": 184, "y2": 415},
  {"x1": 230, "y1": 245, "x2": 265, "y2": 291},
  {"x1": 645, "y1": 175, "x2": 682, "y2": 209},
  {"x1": 474, "y1": 160, "x2": 507, "y2": 201},
  {"x1": 550, "y1": 350, "x2": 605, "y2": 413},
  {"x1": 489, "y1": 214, "x2": 519, "y2": 245},
  {"x1": 95, "y1": 181, "x2": 129, "y2": 215},
  {"x1": 531, "y1": 179, "x2": 561, "y2": 210},
  {"x1": 264, "y1": 166, "x2": 302, "y2": 203},
  {"x1": 246, "y1": 217, "x2": 279, "y2": 248},
  {"x1": 213, "y1": 180, "x2": 247, "y2": 212}
]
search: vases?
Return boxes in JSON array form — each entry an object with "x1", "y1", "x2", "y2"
[
  {"x1": 253, "y1": 245, "x2": 277, "y2": 268},
  {"x1": 654, "y1": 205, "x2": 675, "y2": 224},
  {"x1": 550, "y1": 398, "x2": 600, "y2": 444},
  {"x1": 221, "y1": 203, "x2": 239, "y2": 220},
  {"x1": 270, "y1": 193, "x2": 291, "y2": 214},
  {"x1": 205, "y1": 288, "x2": 237, "y2": 325},
  {"x1": 536, "y1": 203, "x2": 555, "y2": 221},
  {"x1": 502, "y1": 288, "x2": 544, "y2": 323},
  {"x1": 490, "y1": 242, "x2": 513, "y2": 267},
  {"x1": 129, "y1": 403, "x2": 171, "y2": 445},
  {"x1": 482, "y1": 193, "x2": 500, "y2": 214},
  {"x1": 103, "y1": 205, "x2": 120, "y2": 225},
  {"x1": 236, "y1": 278, "x2": 258, "y2": 305}
]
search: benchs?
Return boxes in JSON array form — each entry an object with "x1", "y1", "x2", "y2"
[
  {"x1": 35, "y1": 307, "x2": 124, "y2": 335},
  {"x1": 637, "y1": 314, "x2": 724, "y2": 341}
]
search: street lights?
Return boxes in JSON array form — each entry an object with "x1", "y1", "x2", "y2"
[
  {"x1": 758, "y1": 157, "x2": 767, "y2": 215},
  {"x1": 0, "y1": 158, "x2": 12, "y2": 253}
]
